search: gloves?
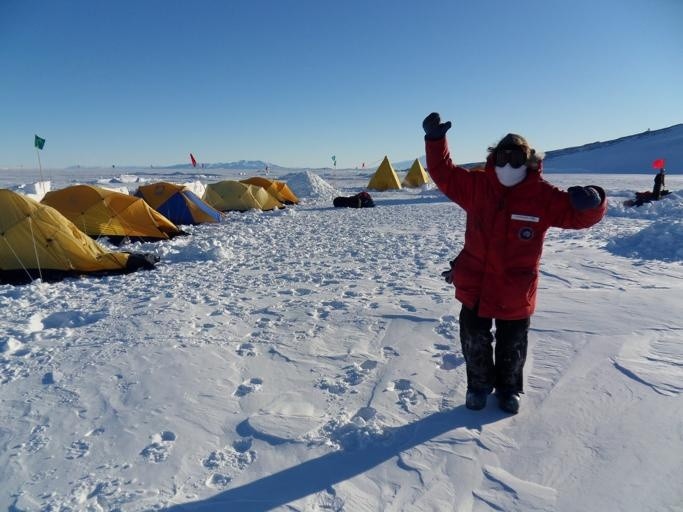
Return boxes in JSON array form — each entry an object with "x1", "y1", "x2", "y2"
[
  {"x1": 422, "y1": 112, "x2": 451, "y2": 140},
  {"x1": 567, "y1": 185, "x2": 600, "y2": 211}
]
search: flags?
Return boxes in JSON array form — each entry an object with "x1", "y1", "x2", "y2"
[
  {"x1": 331, "y1": 155, "x2": 335, "y2": 160},
  {"x1": 652, "y1": 158, "x2": 665, "y2": 168},
  {"x1": 190, "y1": 153, "x2": 196, "y2": 167},
  {"x1": 334, "y1": 161, "x2": 336, "y2": 166},
  {"x1": 34, "y1": 135, "x2": 45, "y2": 150}
]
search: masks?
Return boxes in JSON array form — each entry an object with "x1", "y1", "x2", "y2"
[{"x1": 494, "y1": 163, "x2": 527, "y2": 188}]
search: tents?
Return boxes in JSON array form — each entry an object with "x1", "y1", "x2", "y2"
[
  {"x1": 406, "y1": 158, "x2": 429, "y2": 186},
  {"x1": 134, "y1": 182, "x2": 226, "y2": 225},
  {"x1": 240, "y1": 177, "x2": 300, "y2": 203},
  {"x1": 1, "y1": 188, "x2": 159, "y2": 286},
  {"x1": 39, "y1": 184, "x2": 192, "y2": 241},
  {"x1": 367, "y1": 154, "x2": 401, "y2": 189},
  {"x1": 202, "y1": 180, "x2": 286, "y2": 212}
]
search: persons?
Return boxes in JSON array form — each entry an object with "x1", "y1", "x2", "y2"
[
  {"x1": 653, "y1": 168, "x2": 665, "y2": 201},
  {"x1": 423, "y1": 113, "x2": 607, "y2": 413}
]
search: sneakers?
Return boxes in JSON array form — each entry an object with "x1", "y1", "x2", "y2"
[
  {"x1": 495, "y1": 389, "x2": 518, "y2": 414},
  {"x1": 465, "y1": 389, "x2": 486, "y2": 409}
]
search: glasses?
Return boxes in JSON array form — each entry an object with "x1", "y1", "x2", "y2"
[{"x1": 494, "y1": 149, "x2": 526, "y2": 168}]
